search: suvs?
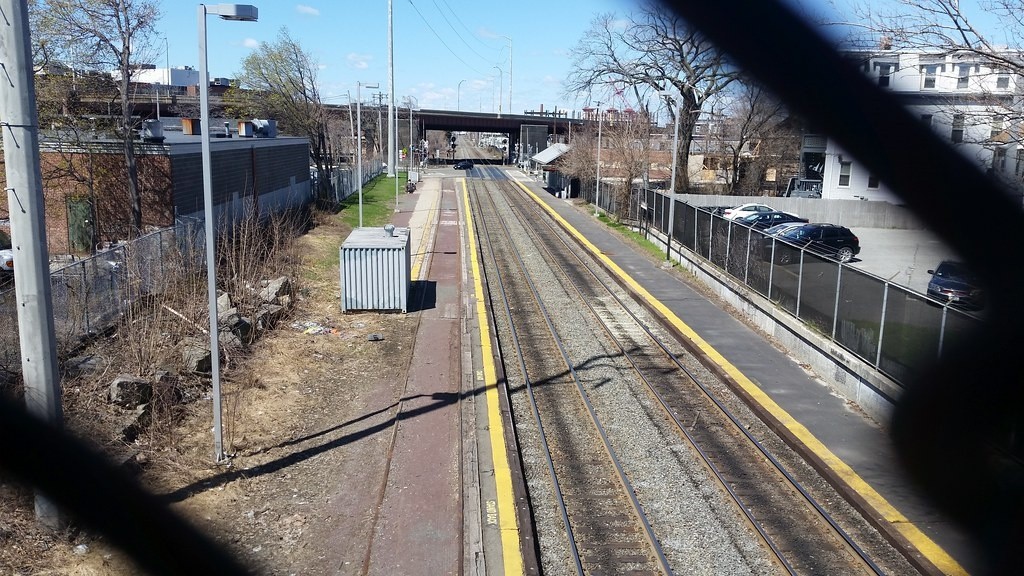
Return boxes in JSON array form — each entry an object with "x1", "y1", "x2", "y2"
[{"x1": 758, "y1": 222, "x2": 861, "y2": 265}]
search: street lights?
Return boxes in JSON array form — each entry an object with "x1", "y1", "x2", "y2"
[
  {"x1": 457, "y1": 79, "x2": 467, "y2": 111},
  {"x1": 492, "y1": 66, "x2": 503, "y2": 119},
  {"x1": 496, "y1": 33, "x2": 515, "y2": 114},
  {"x1": 164, "y1": 36, "x2": 172, "y2": 98},
  {"x1": 582, "y1": 106, "x2": 601, "y2": 217},
  {"x1": 402, "y1": 100, "x2": 414, "y2": 171},
  {"x1": 657, "y1": 90, "x2": 679, "y2": 241},
  {"x1": 196, "y1": 2, "x2": 259, "y2": 463},
  {"x1": 356, "y1": 80, "x2": 380, "y2": 228}
]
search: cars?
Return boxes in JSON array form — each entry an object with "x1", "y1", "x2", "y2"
[
  {"x1": 723, "y1": 204, "x2": 799, "y2": 224},
  {"x1": 454, "y1": 162, "x2": 474, "y2": 171},
  {"x1": 927, "y1": 259, "x2": 981, "y2": 310},
  {"x1": 727, "y1": 213, "x2": 810, "y2": 247},
  {"x1": 743, "y1": 223, "x2": 818, "y2": 253}
]
